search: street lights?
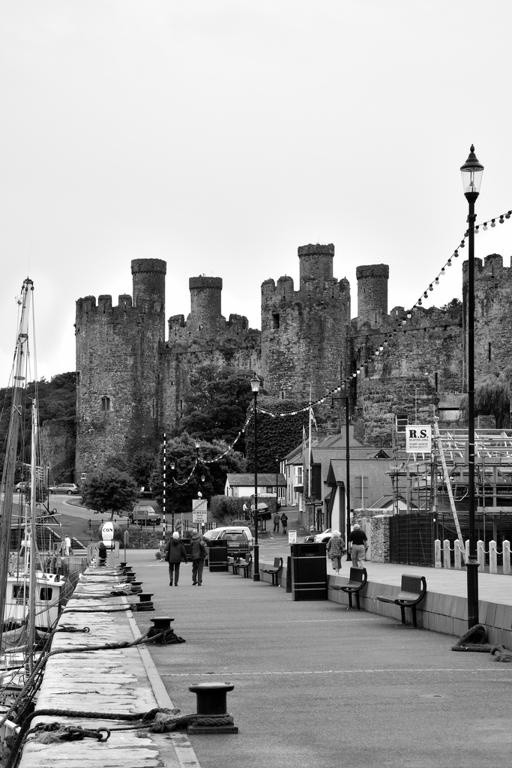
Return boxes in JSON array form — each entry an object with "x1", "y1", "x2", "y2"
[
  {"x1": 194, "y1": 440, "x2": 200, "y2": 496},
  {"x1": 458, "y1": 145, "x2": 487, "y2": 633},
  {"x1": 247, "y1": 371, "x2": 262, "y2": 581}
]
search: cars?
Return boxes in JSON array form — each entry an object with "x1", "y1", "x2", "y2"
[{"x1": 249, "y1": 502, "x2": 271, "y2": 520}]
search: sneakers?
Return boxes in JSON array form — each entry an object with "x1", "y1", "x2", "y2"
[
  {"x1": 192, "y1": 581, "x2": 201, "y2": 585},
  {"x1": 169, "y1": 582, "x2": 177, "y2": 586}
]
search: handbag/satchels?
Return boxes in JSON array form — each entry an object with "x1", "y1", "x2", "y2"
[{"x1": 165, "y1": 551, "x2": 170, "y2": 561}]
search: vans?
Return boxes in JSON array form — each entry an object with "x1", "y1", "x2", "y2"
[{"x1": 202, "y1": 526, "x2": 254, "y2": 557}]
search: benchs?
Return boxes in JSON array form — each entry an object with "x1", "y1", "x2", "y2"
[
  {"x1": 376, "y1": 574, "x2": 426, "y2": 627},
  {"x1": 329, "y1": 567, "x2": 367, "y2": 610},
  {"x1": 237, "y1": 554, "x2": 252, "y2": 578},
  {"x1": 227, "y1": 552, "x2": 240, "y2": 574},
  {"x1": 260, "y1": 557, "x2": 283, "y2": 586}
]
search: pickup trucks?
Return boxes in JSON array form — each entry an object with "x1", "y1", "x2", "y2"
[{"x1": 129, "y1": 505, "x2": 162, "y2": 525}]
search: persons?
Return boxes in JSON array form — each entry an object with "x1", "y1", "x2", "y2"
[
  {"x1": 190, "y1": 534, "x2": 210, "y2": 586},
  {"x1": 166, "y1": 531, "x2": 188, "y2": 586},
  {"x1": 327, "y1": 530, "x2": 345, "y2": 575},
  {"x1": 243, "y1": 501, "x2": 250, "y2": 520},
  {"x1": 348, "y1": 524, "x2": 367, "y2": 569},
  {"x1": 281, "y1": 513, "x2": 288, "y2": 534},
  {"x1": 273, "y1": 512, "x2": 280, "y2": 534},
  {"x1": 97, "y1": 542, "x2": 107, "y2": 566}
]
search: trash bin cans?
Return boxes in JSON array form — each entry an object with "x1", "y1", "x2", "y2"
[
  {"x1": 181, "y1": 538, "x2": 193, "y2": 562},
  {"x1": 291, "y1": 542, "x2": 328, "y2": 601},
  {"x1": 208, "y1": 540, "x2": 228, "y2": 572}
]
